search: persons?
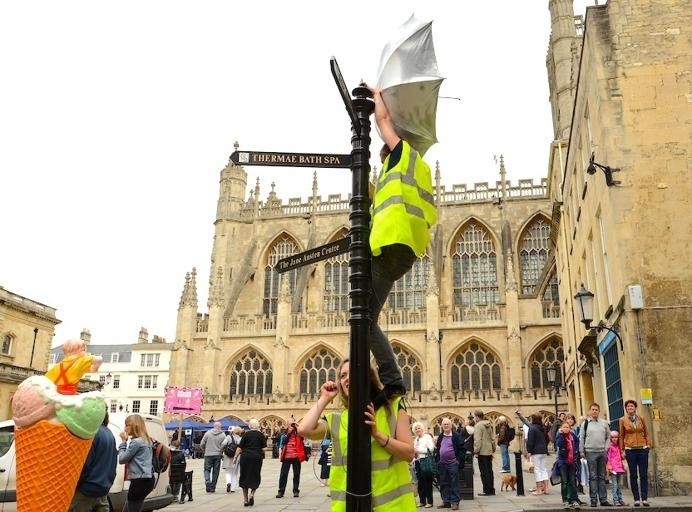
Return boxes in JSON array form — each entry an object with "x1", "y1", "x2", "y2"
[
  {"x1": 366, "y1": 87, "x2": 436, "y2": 407},
  {"x1": 200, "y1": 419, "x2": 267, "y2": 507},
  {"x1": 318, "y1": 438, "x2": 332, "y2": 487},
  {"x1": 69, "y1": 403, "x2": 155, "y2": 512},
  {"x1": 44, "y1": 336, "x2": 102, "y2": 395},
  {"x1": 299, "y1": 357, "x2": 416, "y2": 511},
  {"x1": 495, "y1": 416, "x2": 510, "y2": 474},
  {"x1": 169, "y1": 440, "x2": 186, "y2": 502},
  {"x1": 408, "y1": 408, "x2": 496, "y2": 511},
  {"x1": 172, "y1": 430, "x2": 204, "y2": 459},
  {"x1": 515, "y1": 399, "x2": 651, "y2": 510},
  {"x1": 271, "y1": 426, "x2": 288, "y2": 458},
  {"x1": 275, "y1": 422, "x2": 305, "y2": 498}
]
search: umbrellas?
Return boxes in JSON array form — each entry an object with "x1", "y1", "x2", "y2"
[{"x1": 376, "y1": 12, "x2": 459, "y2": 159}]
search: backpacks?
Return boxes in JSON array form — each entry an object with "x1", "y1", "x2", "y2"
[
  {"x1": 151, "y1": 437, "x2": 171, "y2": 473},
  {"x1": 506, "y1": 425, "x2": 515, "y2": 442},
  {"x1": 225, "y1": 435, "x2": 237, "y2": 457}
]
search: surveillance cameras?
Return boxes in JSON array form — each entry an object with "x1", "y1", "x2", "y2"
[{"x1": 587, "y1": 164, "x2": 596, "y2": 174}]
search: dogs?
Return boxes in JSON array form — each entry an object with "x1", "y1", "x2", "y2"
[{"x1": 500, "y1": 473, "x2": 516, "y2": 492}]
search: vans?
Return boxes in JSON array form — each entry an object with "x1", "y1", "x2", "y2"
[{"x1": 0, "y1": 414, "x2": 174, "y2": 511}]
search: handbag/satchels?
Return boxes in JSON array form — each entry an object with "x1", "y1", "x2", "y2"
[{"x1": 420, "y1": 449, "x2": 436, "y2": 478}]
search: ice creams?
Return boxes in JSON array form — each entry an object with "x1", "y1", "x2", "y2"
[{"x1": 11, "y1": 375, "x2": 108, "y2": 512}]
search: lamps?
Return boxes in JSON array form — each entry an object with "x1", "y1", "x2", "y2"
[
  {"x1": 545, "y1": 364, "x2": 567, "y2": 393},
  {"x1": 573, "y1": 283, "x2": 624, "y2": 352}
]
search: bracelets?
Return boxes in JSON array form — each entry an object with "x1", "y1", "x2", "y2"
[{"x1": 380, "y1": 434, "x2": 390, "y2": 447}]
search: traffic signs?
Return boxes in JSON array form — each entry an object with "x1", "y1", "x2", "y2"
[
  {"x1": 228, "y1": 150, "x2": 356, "y2": 169},
  {"x1": 330, "y1": 56, "x2": 363, "y2": 136},
  {"x1": 269, "y1": 235, "x2": 351, "y2": 273}
]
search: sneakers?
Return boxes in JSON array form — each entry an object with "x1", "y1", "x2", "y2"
[
  {"x1": 564, "y1": 500, "x2": 650, "y2": 510},
  {"x1": 276, "y1": 492, "x2": 284, "y2": 498},
  {"x1": 206, "y1": 481, "x2": 216, "y2": 492},
  {"x1": 294, "y1": 492, "x2": 299, "y2": 497},
  {"x1": 417, "y1": 502, "x2": 458, "y2": 509}
]
